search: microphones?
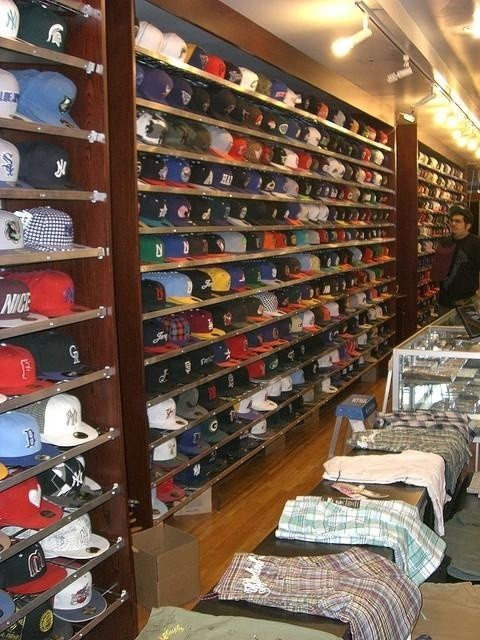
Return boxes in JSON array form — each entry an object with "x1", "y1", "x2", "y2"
[{"x1": 328, "y1": 393, "x2": 377, "y2": 460}]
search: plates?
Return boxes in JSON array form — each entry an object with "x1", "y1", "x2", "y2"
[
  {"x1": 397, "y1": 139, "x2": 469, "y2": 333},
  {"x1": 390, "y1": 306, "x2": 479, "y2": 494},
  {"x1": 1, "y1": 1, "x2": 135, "y2": 639},
  {"x1": 124, "y1": 46, "x2": 401, "y2": 527}
]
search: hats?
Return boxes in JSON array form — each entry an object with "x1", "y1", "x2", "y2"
[
  {"x1": 2, "y1": 279, "x2": 49, "y2": 328},
  {"x1": 2, "y1": 0, "x2": 21, "y2": 40},
  {"x1": 2, "y1": 411, "x2": 60, "y2": 466},
  {"x1": 37, "y1": 454, "x2": 103, "y2": 509},
  {"x1": 2, "y1": 594, "x2": 73, "y2": 638},
  {"x1": 1, "y1": 508, "x2": 108, "y2": 560},
  {"x1": 132, "y1": 20, "x2": 394, "y2": 522},
  {"x1": 417, "y1": 153, "x2": 465, "y2": 328},
  {"x1": 0, "y1": 70, "x2": 31, "y2": 123},
  {"x1": 20, "y1": 141, "x2": 81, "y2": 190},
  {"x1": 13, "y1": 71, "x2": 81, "y2": 126},
  {"x1": 0, "y1": 139, "x2": 34, "y2": 189},
  {"x1": 1, "y1": 342, "x2": 53, "y2": 395},
  {"x1": 48, "y1": 558, "x2": 107, "y2": 623},
  {"x1": 5, "y1": 270, "x2": 91, "y2": 318},
  {"x1": 22, "y1": 206, "x2": 77, "y2": 251},
  {"x1": 2, "y1": 467, "x2": 63, "y2": 529},
  {"x1": 17, "y1": 393, "x2": 99, "y2": 446},
  {"x1": 1, "y1": 209, "x2": 37, "y2": 255},
  {"x1": 2, "y1": 538, "x2": 68, "y2": 595},
  {"x1": 20, "y1": 331, "x2": 93, "y2": 380},
  {"x1": 16, "y1": 2, "x2": 66, "y2": 53}
]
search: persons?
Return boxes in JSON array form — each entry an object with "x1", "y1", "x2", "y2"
[{"x1": 433, "y1": 204, "x2": 480, "y2": 320}]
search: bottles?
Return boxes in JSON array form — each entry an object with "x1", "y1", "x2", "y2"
[{"x1": 417, "y1": 153, "x2": 465, "y2": 328}]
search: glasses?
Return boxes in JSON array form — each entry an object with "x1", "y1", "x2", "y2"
[
  {"x1": 330, "y1": 18, "x2": 372, "y2": 60},
  {"x1": 386, "y1": 55, "x2": 414, "y2": 83}
]
